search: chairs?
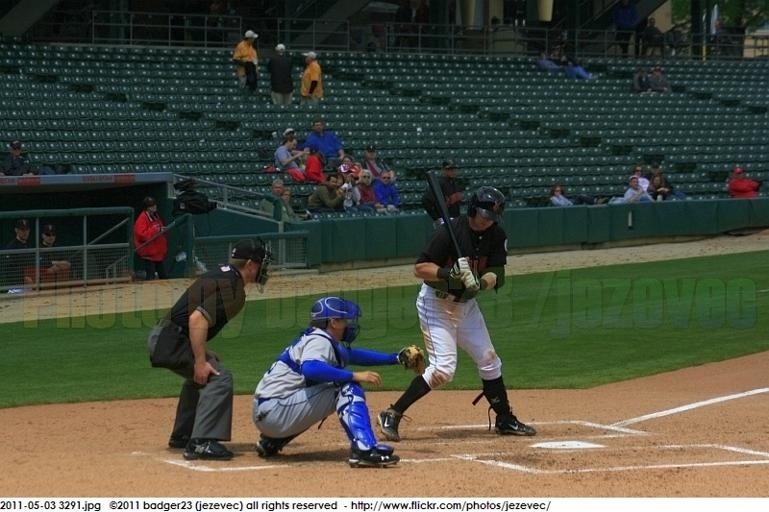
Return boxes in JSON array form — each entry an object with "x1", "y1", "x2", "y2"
[{"x1": 0, "y1": 42, "x2": 769, "y2": 220}]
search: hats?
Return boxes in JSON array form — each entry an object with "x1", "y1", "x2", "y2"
[
  {"x1": 15, "y1": 220, "x2": 29, "y2": 229},
  {"x1": 245, "y1": 30, "x2": 258, "y2": 38},
  {"x1": 144, "y1": 197, "x2": 155, "y2": 207},
  {"x1": 275, "y1": 44, "x2": 285, "y2": 51},
  {"x1": 735, "y1": 167, "x2": 742, "y2": 173},
  {"x1": 301, "y1": 52, "x2": 316, "y2": 60},
  {"x1": 43, "y1": 225, "x2": 55, "y2": 234}
]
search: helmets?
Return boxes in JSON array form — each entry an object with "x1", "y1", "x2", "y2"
[
  {"x1": 311, "y1": 297, "x2": 361, "y2": 343},
  {"x1": 232, "y1": 239, "x2": 272, "y2": 285},
  {"x1": 467, "y1": 186, "x2": 505, "y2": 222}
]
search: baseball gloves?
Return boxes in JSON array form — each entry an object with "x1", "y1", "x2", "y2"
[{"x1": 398, "y1": 344, "x2": 427, "y2": 376}]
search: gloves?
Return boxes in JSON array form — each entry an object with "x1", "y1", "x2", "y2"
[{"x1": 449, "y1": 257, "x2": 480, "y2": 291}]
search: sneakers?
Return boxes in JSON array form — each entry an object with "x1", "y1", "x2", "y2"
[
  {"x1": 495, "y1": 416, "x2": 535, "y2": 436},
  {"x1": 169, "y1": 436, "x2": 233, "y2": 460},
  {"x1": 257, "y1": 440, "x2": 278, "y2": 456},
  {"x1": 350, "y1": 448, "x2": 399, "y2": 468},
  {"x1": 377, "y1": 407, "x2": 399, "y2": 441}
]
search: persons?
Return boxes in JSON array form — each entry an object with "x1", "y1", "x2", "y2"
[
  {"x1": 268, "y1": 43, "x2": 295, "y2": 106},
  {"x1": 544, "y1": 162, "x2": 686, "y2": 208},
  {"x1": 232, "y1": 30, "x2": 261, "y2": 89},
  {"x1": 377, "y1": 185, "x2": 536, "y2": 440},
  {"x1": 269, "y1": 117, "x2": 467, "y2": 224},
  {"x1": 536, "y1": 17, "x2": 727, "y2": 95},
  {"x1": 728, "y1": 167, "x2": 759, "y2": 199},
  {"x1": 300, "y1": 51, "x2": 324, "y2": 102},
  {"x1": 0, "y1": 195, "x2": 168, "y2": 291},
  {"x1": 147, "y1": 238, "x2": 273, "y2": 460},
  {"x1": 251, "y1": 295, "x2": 425, "y2": 468},
  {"x1": 3, "y1": 140, "x2": 39, "y2": 177}
]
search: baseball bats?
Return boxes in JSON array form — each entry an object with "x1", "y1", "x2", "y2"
[{"x1": 427, "y1": 171, "x2": 476, "y2": 290}]
